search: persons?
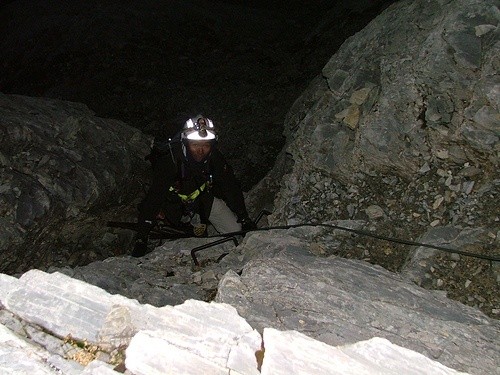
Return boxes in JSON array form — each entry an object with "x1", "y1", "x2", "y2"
[{"x1": 142, "y1": 112, "x2": 258, "y2": 238}]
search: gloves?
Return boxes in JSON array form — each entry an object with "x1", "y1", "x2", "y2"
[
  {"x1": 130, "y1": 238, "x2": 147, "y2": 258},
  {"x1": 236, "y1": 216, "x2": 256, "y2": 236}
]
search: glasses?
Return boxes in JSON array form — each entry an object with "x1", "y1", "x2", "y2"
[{"x1": 186, "y1": 142, "x2": 213, "y2": 149}]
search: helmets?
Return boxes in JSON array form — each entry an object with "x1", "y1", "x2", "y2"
[{"x1": 182, "y1": 115, "x2": 218, "y2": 141}]
